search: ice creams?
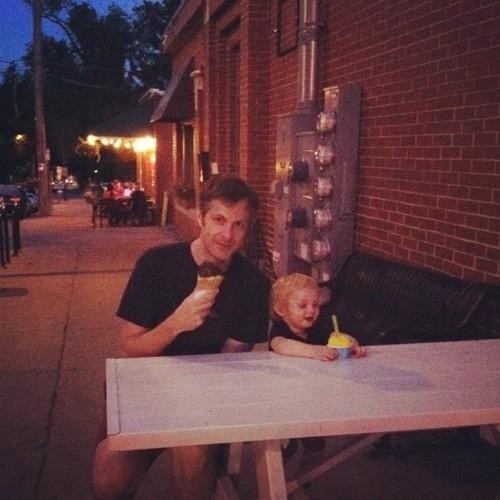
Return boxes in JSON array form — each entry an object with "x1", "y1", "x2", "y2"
[
  {"x1": 196, "y1": 261, "x2": 223, "y2": 290},
  {"x1": 328, "y1": 331, "x2": 353, "y2": 349}
]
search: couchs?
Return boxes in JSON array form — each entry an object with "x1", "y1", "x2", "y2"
[{"x1": 317, "y1": 248, "x2": 500, "y2": 338}]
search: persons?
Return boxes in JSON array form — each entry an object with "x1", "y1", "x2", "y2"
[
  {"x1": 266, "y1": 271, "x2": 369, "y2": 492},
  {"x1": 91, "y1": 175, "x2": 147, "y2": 229},
  {"x1": 91, "y1": 174, "x2": 272, "y2": 500}
]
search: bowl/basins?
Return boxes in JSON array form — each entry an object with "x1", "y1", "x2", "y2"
[{"x1": 326, "y1": 345, "x2": 354, "y2": 360}]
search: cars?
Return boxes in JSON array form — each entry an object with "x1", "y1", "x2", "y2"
[{"x1": 0, "y1": 184, "x2": 39, "y2": 219}]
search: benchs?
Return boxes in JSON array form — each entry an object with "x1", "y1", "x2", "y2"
[{"x1": 92, "y1": 197, "x2": 161, "y2": 228}]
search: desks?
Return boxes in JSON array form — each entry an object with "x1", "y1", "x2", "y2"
[{"x1": 104, "y1": 338, "x2": 500, "y2": 500}]
